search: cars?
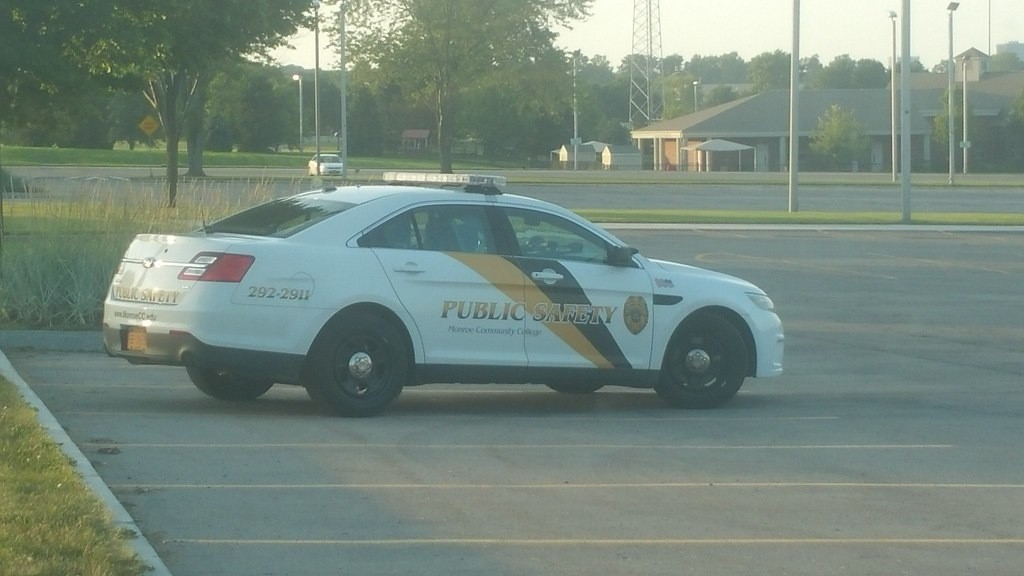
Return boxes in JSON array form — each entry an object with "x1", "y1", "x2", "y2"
[
  {"x1": 309, "y1": 154, "x2": 344, "y2": 177},
  {"x1": 100, "y1": 170, "x2": 785, "y2": 416}
]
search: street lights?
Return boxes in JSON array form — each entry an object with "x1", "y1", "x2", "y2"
[
  {"x1": 901, "y1": 0, "x2": 911, "y2": 223},
  {"x1": 887, "y1": 10, "x2": 898, "y2": 182},
  {"x1": 945, "y1": 2, "x2": 959, "y2": 185},
  {"x1": 693, "y1": 80, "x2": 700, "y2": 111},
  {"x1": 310, "y1": 0, "x2": 321, "y2": 174},
  {"x1": 293, "y1": 74, "x2": 304, "y2": 155},
  {"x1": 787, "y1": 0, "x2": 800, "y2": 212},
  {"x1": 961, "y1": 56, "x2": 972, "y2": 175}
]
